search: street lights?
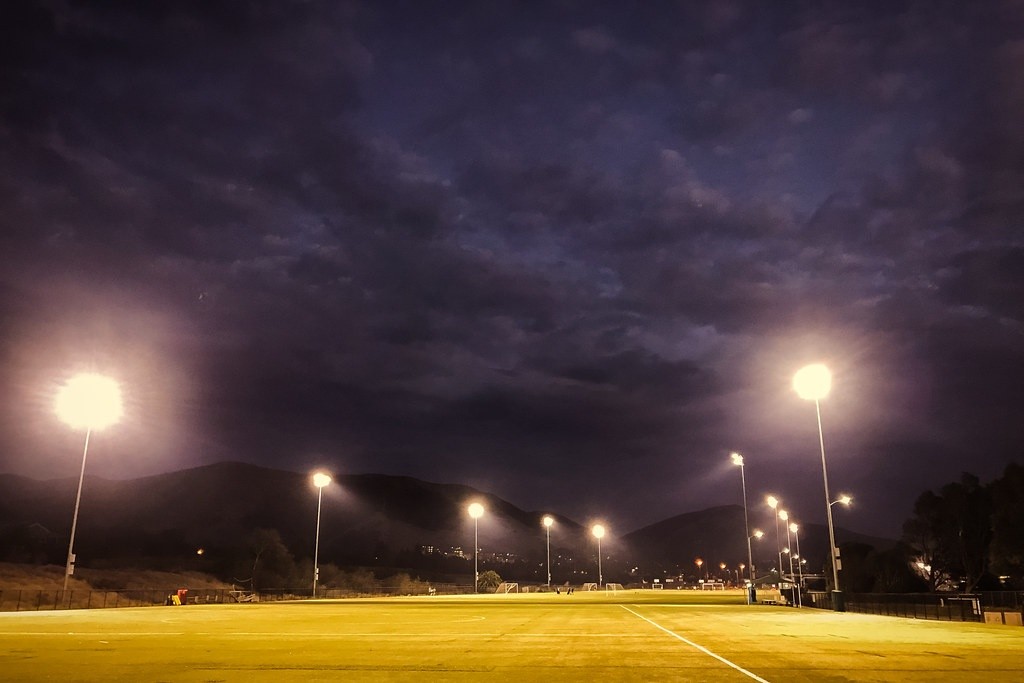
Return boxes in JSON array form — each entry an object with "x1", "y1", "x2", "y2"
[
  {"x1": 469, "y1": 504, "x2": 483, "y2": 593},
  {"x1": 593, "y1": 525, "x2": 604, "y2": 587},
  {"x1": 731, "y1": 453, "x2": 764, "y2": 606},
  {"x1": 778, "y1": 509, "x2": 800, "y2": 576},
  {"x1": 58, "y1": 376, "x2": 118, "y2": 609},
  {"x1": 767, "y1": 497, "x2": 789, "y2": 580},
  {"x1": 309, "y1": 473, "x2": 331, "y2": 600},
  {"x1": 794, "y1": 364, "x2": 854, "y2": 612},
  {"x1": 790, "y1": 522, "x2": 806, "y2": 576},
  {"x1": 543, "y1": 517, "x2": 553, "y2": 591}
]
